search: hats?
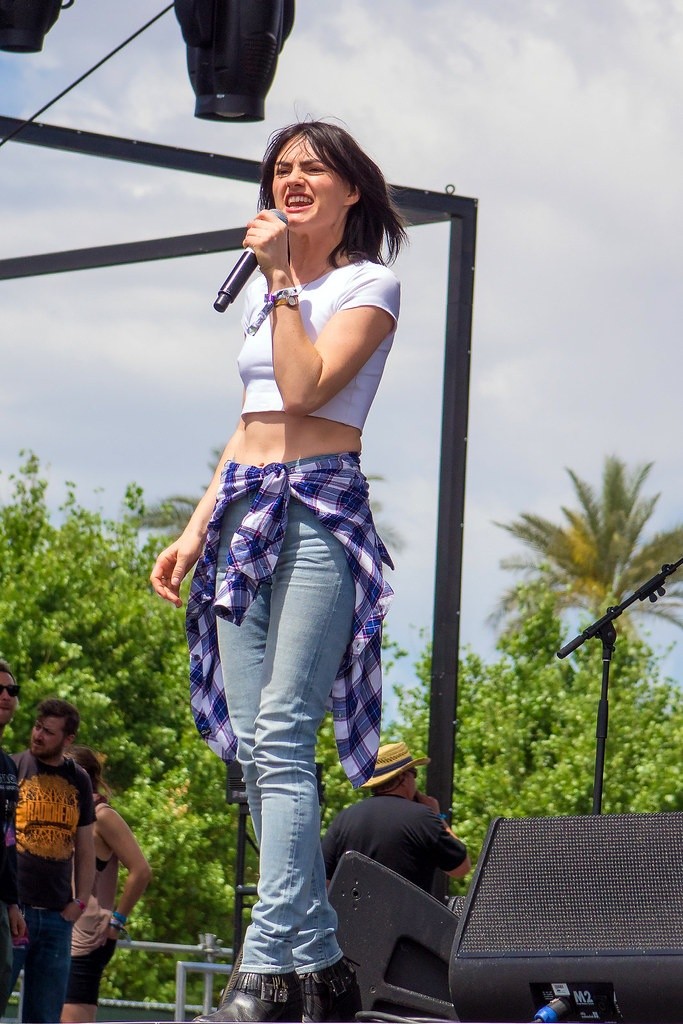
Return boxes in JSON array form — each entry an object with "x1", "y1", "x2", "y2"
[{"x1": 360, "y1": 741, "x2": 431, "y2": 788}]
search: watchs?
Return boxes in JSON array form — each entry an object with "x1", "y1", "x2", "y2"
[{"x1": 275, "y1": 296, "x2": 299, "y2": 306}]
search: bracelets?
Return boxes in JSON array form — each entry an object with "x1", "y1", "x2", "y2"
[
  {"x1": 247, "y1": 287, "x2": 299, "y2": 336},
  {"x1": 112, "y1": 912, "x2": 127, "y2": 923},
  {"x1": 109, "y1": 919, "x2": 123, "y2": 930},
  {"x1": 74, "y1": 899, "x2": 87, "y2": 912},
  {"x1": 437, "y1": 813, "x2": 446, "y2": 820}
]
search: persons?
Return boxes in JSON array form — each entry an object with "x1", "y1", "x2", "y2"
[
  {"x1": 0, "y1": 661, "x2": 28, "y2": 1015},
  {"x1": 60, "y1": 746, "x2": 150, "y2": 1022},
  {"x1": 0, "y1": 700, "x2": 98, "y2": 1024},
  {"x1": 147, "y1": 122, "x2": 405, "y2": 1022},
  {"x1": 321, "y1": 742, "x2": 472, "y2": 894}
]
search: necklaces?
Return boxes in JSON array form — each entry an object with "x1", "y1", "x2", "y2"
[{"x1": 299, "y1": 266, "x2": 329, "y2": 293}]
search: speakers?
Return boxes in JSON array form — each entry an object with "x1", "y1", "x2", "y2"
[
  {"x1": 327, "y1": 850, "x2": 461, "y2": 1023},
  {"x1": 448, "y1": 813, "x2": 683, "y2": 1024}
]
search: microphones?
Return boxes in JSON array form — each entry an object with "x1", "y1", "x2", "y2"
[{"x1": 213, "y1": 209, "x2": 288, "y2": 313}]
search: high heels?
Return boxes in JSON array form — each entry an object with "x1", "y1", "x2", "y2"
[
  {"x1": 299, "y1": 953, "x2": 363, "y2": 1023},
  {"x1": 192, "y1": 966, "x2": 302, "y2": 1023}
]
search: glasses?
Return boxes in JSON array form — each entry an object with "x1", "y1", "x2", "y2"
[
  {"x1": 0, "y1": 683, "x2": 20, "y2": 697},
  {"x1": 406, "y1": 768, "x2": 417, "y2": 779}
]
